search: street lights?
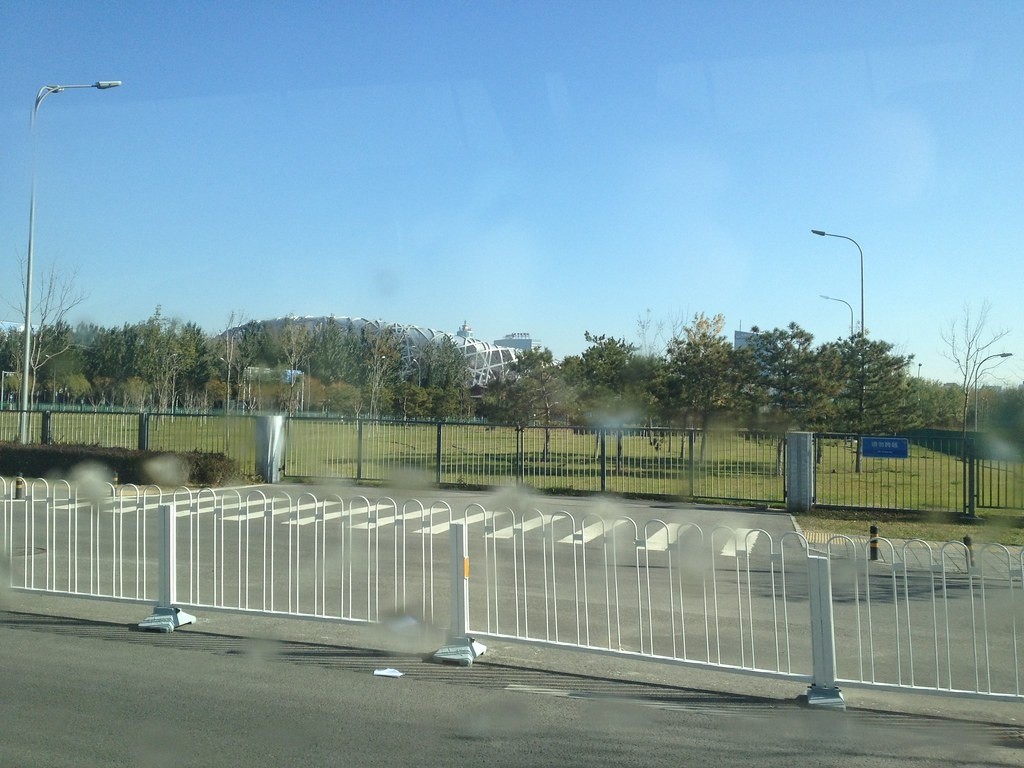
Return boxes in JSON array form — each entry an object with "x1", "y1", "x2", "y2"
[
  {"x1": 975, "y1": 353, "x2": 1014, "y2": 432},
  {"x1": 811, "y1": 229, "x2": 865, "y2": 473},
  {"x1": 918, "y1": 362, "x2": 923, "y2": 406},
  {"x1": 45, "y1": 354, "x2": 57, "y2": 402},
  {"x1": 412, "y1": 358, "x2": 421, "y2": 387},
  {"x1": 819, "y1": 294, "x2": 854, "y2": 351},
  {"x1": 377, "y1": 355, "x2": 385, "y2": 378},
  {"x1": 167, "y1": 354, "x2": 178, "y2": 363},
  {"x1": 219, "y1": 358, "x2": 229, "y2": 367}
]
